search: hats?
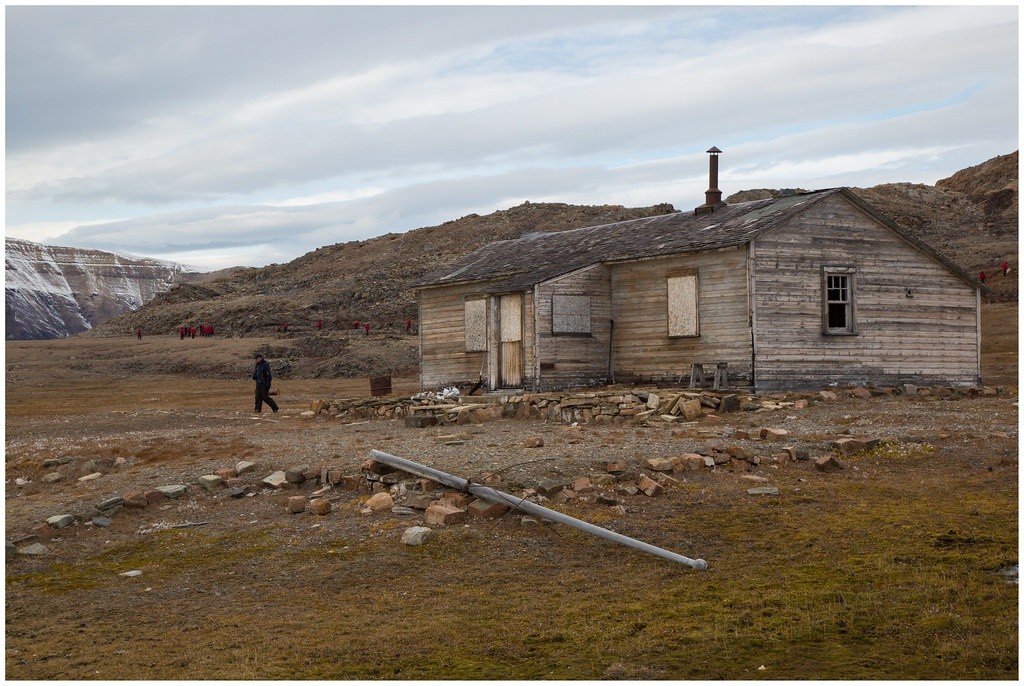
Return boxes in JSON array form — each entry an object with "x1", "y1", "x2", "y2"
[{"x1": 253, "y1": 354, "x2": 261, "y2": 359}]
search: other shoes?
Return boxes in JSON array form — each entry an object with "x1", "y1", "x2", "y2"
[
  {"x1": 273, "y1": 408, "x2": 278, "y2": 414},
  {"x1": 254, "y1": 409, "x2": 261, "y2": 413}
]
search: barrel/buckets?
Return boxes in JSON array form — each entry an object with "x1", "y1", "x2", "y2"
[{"x1": 370, "y1": 375, "x2": 392, "y2": 396}]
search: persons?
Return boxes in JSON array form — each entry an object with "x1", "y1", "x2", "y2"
[
  {"x1": 251, "y1": 353, "x2": 279, "y2": 413},
  {"x1": 364, "y1": 323, "x2": 371, "y2": 335},
  {"x1": 310, "y1": 317, "x2": 322, "y2": 330},
  {"x1": 282, "y1": 320, "x2": 289, "y2": 333},
  {"x1": 980, "y1": 271, "x2": 987, "y2": 283},
  {"x1": 1001, "y1": 256, "x2": 1009, "y2": 277},
  {"x1": 136, "y1": 326, "x2": 142, "y2": 340},
  {"x1": 353, "y1": 319, "x2": 361, "y2": 330},
  {"x1": 405, "y1": 317, "x2": 412, "y2": 335},
  {"x1": 179, "y1": 321, "x2": 215, "y2": 340}
]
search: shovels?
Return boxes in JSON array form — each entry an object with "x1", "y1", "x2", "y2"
[
  {"x1": 468, "y1": 351, "x2": 487, "y2": 396},
  {"x1": 605, "y1": 319, "x2": 614, "y2": 388}
]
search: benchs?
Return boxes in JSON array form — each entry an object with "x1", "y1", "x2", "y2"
[{"x1": 689, "y1": 360, "x2": 729, "y2": 391}]
